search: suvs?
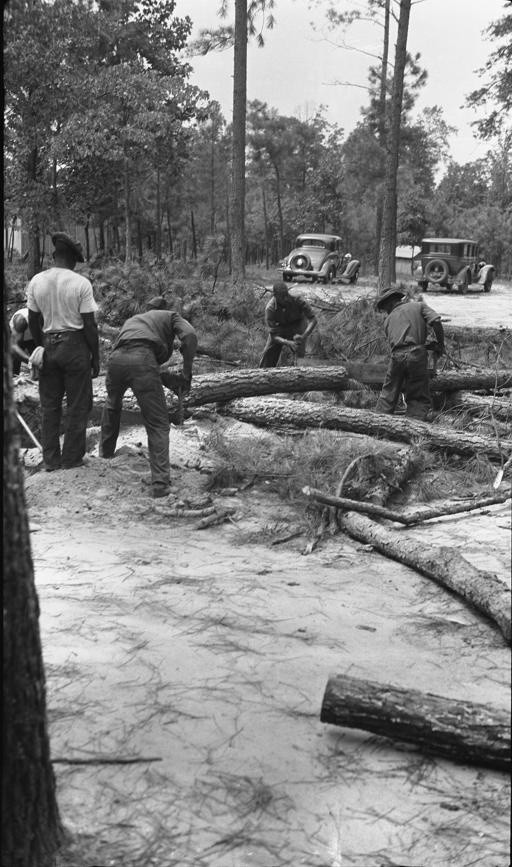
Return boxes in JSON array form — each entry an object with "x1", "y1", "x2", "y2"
[{"x1": 412, "y1": 235, "x2": 496, "y2": 293}]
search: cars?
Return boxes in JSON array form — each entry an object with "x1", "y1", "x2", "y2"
[{"x1": 275, "y1": 231, "x2": 362, "y2": 285}]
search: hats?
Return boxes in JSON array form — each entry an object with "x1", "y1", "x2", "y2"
[
  {"x1": 148, "y1": 298, "x2": 167, "y2": 309},
  {"x1": 52, "y1": 232, "x2": 84, "y2": 263},
  {"x1": 374, "y1": 289, "x2": 405, "y2": 309}
]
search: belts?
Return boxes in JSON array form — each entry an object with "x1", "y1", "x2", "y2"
[{"x1": 46, "y1": 334, "x2": 70, "y2": 340}]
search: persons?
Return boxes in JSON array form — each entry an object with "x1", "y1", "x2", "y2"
[
  {"x1": 375, "y1": 287, "x2": 444, "y2": 420},
  {"x1": 26, "y1": 231, "x2": 99, "y2": 473},
  {"x1": 99, "y1": 296, "x2": 198, "y2": 497},
  {"x1": 9, "y1": 308, "x2": 34, "y2": 375},
  {"x1": 258, "y1": 282, "x2": 317, "y2": 368}
]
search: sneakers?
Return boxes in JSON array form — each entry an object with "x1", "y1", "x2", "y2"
[{"x1": 152, "y1": 482, "x2": 178, "y2": 498}]
